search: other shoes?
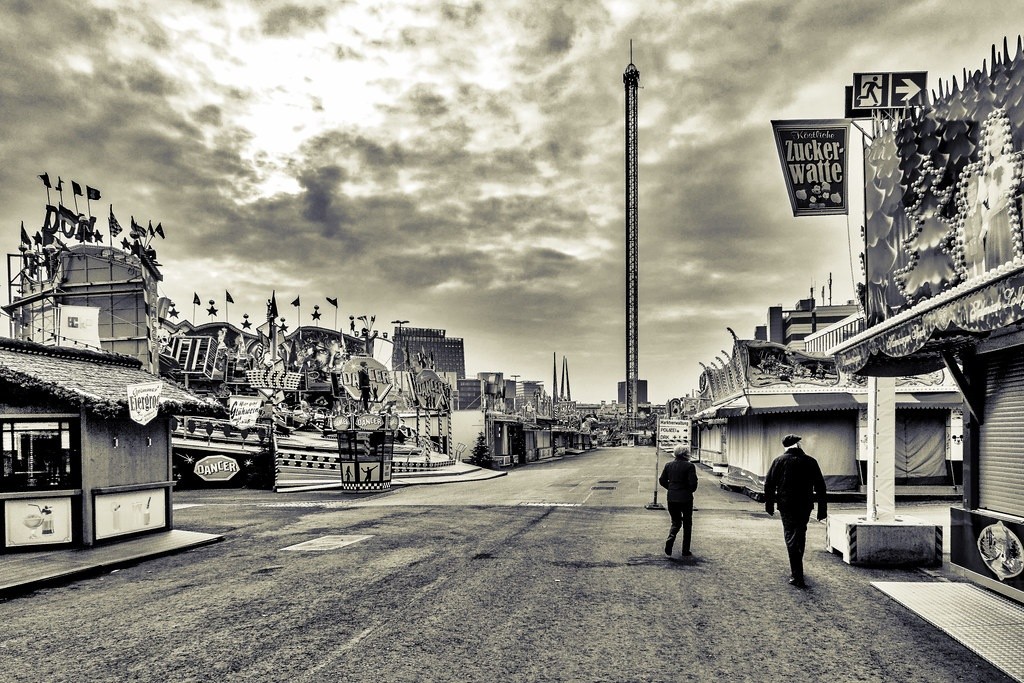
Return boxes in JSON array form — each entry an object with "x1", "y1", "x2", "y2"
[
  {"x1": 788, "y1": 578, "x2": 803, "y2": 585},
  {"x1": 682, "y1": 551, "x2": 692, "y2": 556},
  {"x1": 665, "y1": 539, "x2": 672, "y2": 556}
]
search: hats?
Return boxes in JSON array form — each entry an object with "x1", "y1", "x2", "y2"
[{"x1": 781, "y1": 434, "x2": 802, "y2": 447}]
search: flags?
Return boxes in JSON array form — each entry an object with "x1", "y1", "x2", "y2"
[
  {"x1": 87, "y1": 186, "x2": 101, "y2": 200},
  {"x1": 110, "y1": 213, "x2": 122, "y2": 237},
  {"x1": 72, "y1": 181, "x2": 84, "y2": 196},
  {"x1": 226, "y1": 291, "x2": 234, "y2": 303},
  {"x1": 148, "y1": 224, "x2": 156, "y2": 237},
  {"x1": 156, "y1": 225, "x2": 165, "y2": 239},
  {"x1": 20, "y1": 226, "x2": 32, "y2": 245},
  {"x1": 271, "y1": 296, "x2": 278, "y2": 318},
  {"x1": 326, "y1": 297, "x2": 337, "y2": 307},
  {"x1": 192, "y1": 294, "x2": 201, "y2": 306},
  {"x1": 291, "y1": 297, "x2": 300, "y2": 307},
  {"x1": 40, "y1": 174, "x2": 52, "y2": 189},
  {"x1": 55, "y1": 179, "x2": 64, "y2": 191}
]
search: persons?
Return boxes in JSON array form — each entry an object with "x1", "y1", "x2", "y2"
[
  {"x1": 659, "y1": 445, "x2": 698, "y2": 557},
  {"x1": 358, "y1": 362, "x2": 372, "y2": 415},
  {"x1": 764, "y1": 435, "x2": 828, "y2": 586}
]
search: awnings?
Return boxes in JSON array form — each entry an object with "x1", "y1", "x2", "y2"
[{"x1": 716, "y1": 392, "x2": 963, "y2": 416}]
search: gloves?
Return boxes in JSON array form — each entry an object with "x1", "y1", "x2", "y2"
[
  {"x1": 817, "y1": 513, "x2": 827, "y2": 520},
  {"x1": 765, "y1": 506, "x2": 775, "y2": 516}
]
